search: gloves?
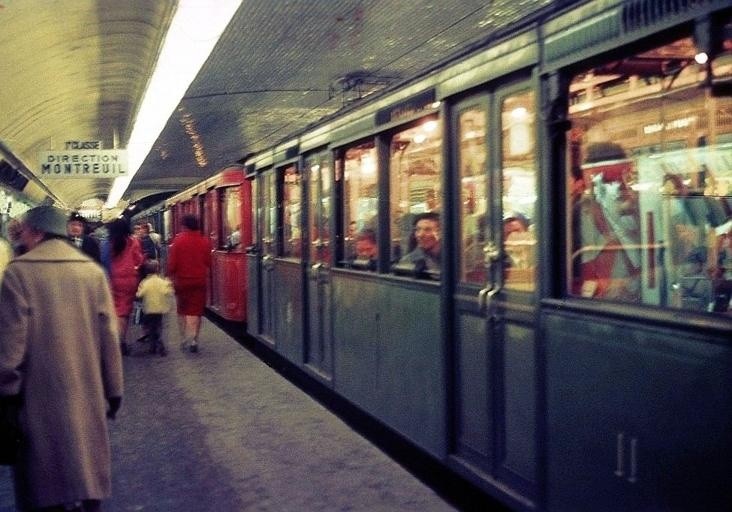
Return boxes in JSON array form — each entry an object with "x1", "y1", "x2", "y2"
[{"x1": 106, "y1": 397, "x2": 120, "y2": 419}]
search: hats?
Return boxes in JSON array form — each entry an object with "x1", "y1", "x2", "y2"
[{"x1": 26, "y1": 206, "x2": 69, "y2": 238}]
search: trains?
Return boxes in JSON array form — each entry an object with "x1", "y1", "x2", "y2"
[{"x1": 132, "y1": 1, "x2": 732, "y2": 512}]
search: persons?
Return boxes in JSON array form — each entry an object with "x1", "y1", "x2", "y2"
[
  {"x1": 1, "y1": 204, "x2": 127, "y2": 512},
  {"x1": 223, "y1": 137, "x2": 731, "y2": 321},
  {"x1": 1, "y1": 210, "x2": 213, "y2": 357}
]
